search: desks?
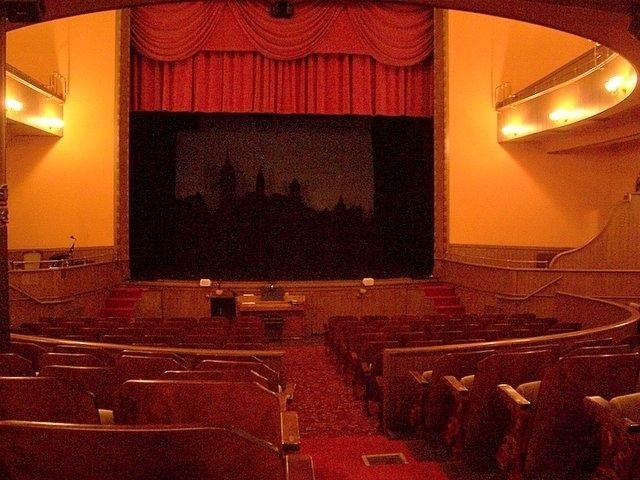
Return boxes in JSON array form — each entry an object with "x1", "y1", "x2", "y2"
[{"x1": 236, "y1": 293, "x2": 308, "y2": 344}]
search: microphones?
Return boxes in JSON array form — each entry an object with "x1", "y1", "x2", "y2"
[{"x1": 70, "y1": 235, "x2": 81, "y2": 256}]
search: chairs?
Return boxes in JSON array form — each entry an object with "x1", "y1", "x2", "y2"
[{"x1": 21, "y1": 249, "x2": 42, "y2": 269}]
